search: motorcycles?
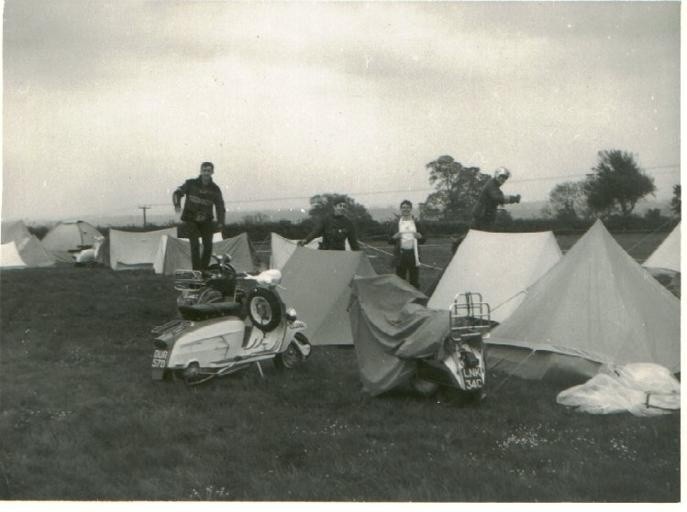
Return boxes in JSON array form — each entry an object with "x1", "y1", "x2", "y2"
[
  {"x1": 347, "y1": 273, "x2": 499, "y2": 402},
  {"x1": 68, "y1": 236, "x2": 106, "y2": 266},
  {"x1": 146, "y1": 267, "x2": 312, "y2": 386},
  {"x1": 172, "y1": 252, "x2": 249, "y2": 318}
]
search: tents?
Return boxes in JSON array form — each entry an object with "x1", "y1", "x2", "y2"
[
  {"x1": 265, "y1": 232, "x2": 359, "y2": 272},
  {"x1": 1, "y1": 217, "x2": 257, "y2": 285},
  {"x1": 426, "y1": 220, "x2": 680, "y2": 376},
  {"x1": 270, "y1": 250, "x2": 381, "y2": 347}
]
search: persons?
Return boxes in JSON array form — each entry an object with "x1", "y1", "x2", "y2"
[
  {"x1": 173, "y1": 162, "x2": 228, "y2": 271},
  {"x1": 472, "y1": 164, "x2": 523, "y2": 231},
  {"x1": 295, "y1": 197, "x2": 362, "y2": 250},
  {"x1": 384, "y1": 199, "x2": 427, "y2": 289}
]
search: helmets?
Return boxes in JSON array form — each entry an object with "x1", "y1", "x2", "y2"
[{"x1": 495, "y1": 166, "x2": 511, "y2": 186}]
